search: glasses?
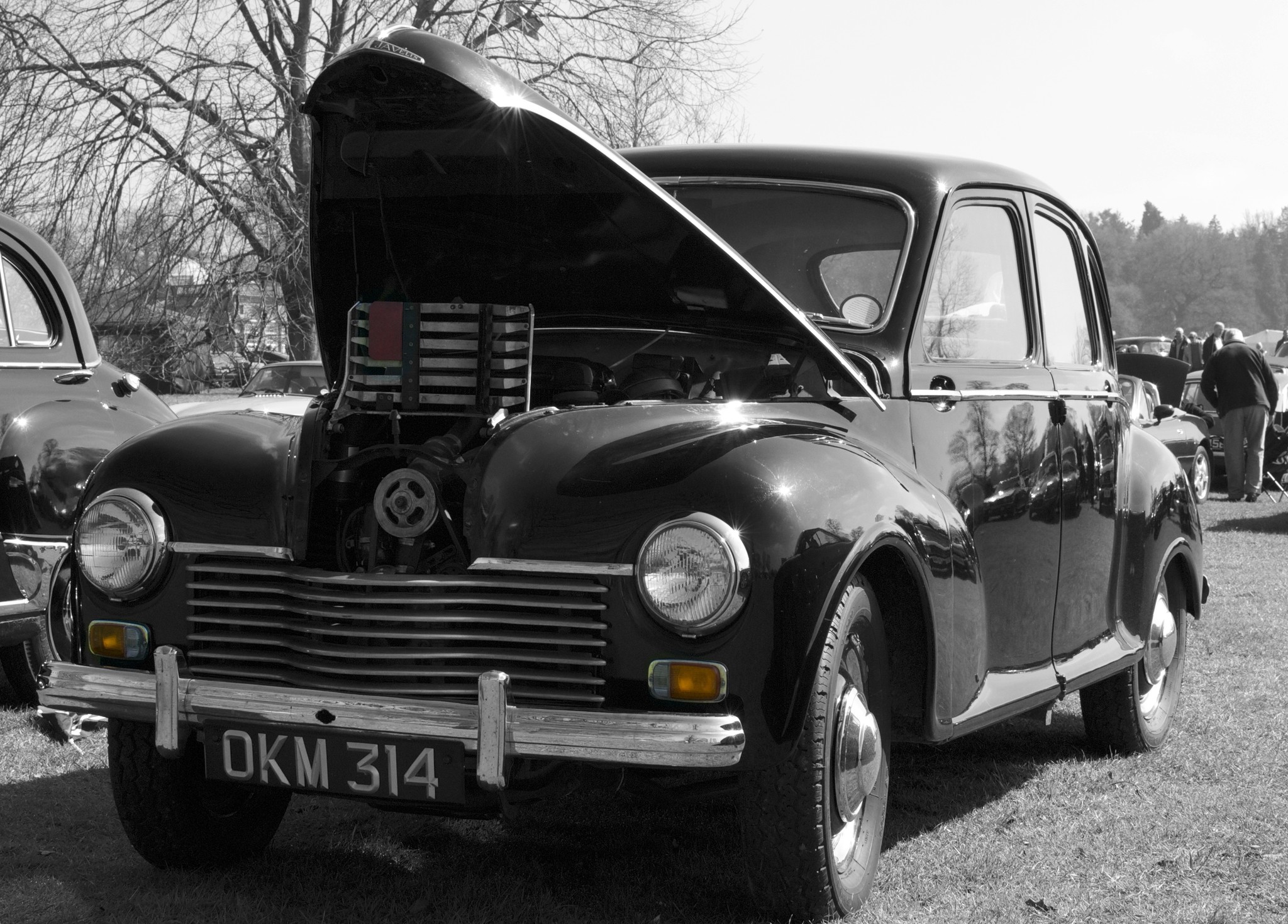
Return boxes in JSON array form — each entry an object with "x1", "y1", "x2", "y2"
[{"x1": 1189, "y1": 336, "x2": 1196, "y2": 339}]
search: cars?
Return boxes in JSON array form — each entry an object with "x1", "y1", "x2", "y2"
[
  {"x1": 38, "y1": 28, "x2": 1214, "y2": 924},
  {"x1": 1113, "y1": 336, "x2": 1287, "y2": 492},
  {"x1": 0, "y1": 212, "x2": 291, "y2": 742},
  {"x1": 168, "y1": 361, "x2": 326, "y2": 418}
]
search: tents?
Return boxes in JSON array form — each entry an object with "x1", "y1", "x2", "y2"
[{"x1": 1244, "y1": 329, "x2": 1283, "y2": 356}]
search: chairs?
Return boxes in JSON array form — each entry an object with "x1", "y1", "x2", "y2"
[{"x1": 1242, "y1": 423, "x2": 1288, "y2": 504}]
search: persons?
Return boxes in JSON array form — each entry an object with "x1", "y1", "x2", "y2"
[
  {"x1": 1201, "y1": 328, "x2": 1279, "y2": 502},
  {"x1": 1275, "y1": 329, "x2": 1288, "y2": 354},
  {"x1": 1167, "y1": 322, "x2": 1230, "y2": 374},
  {"x1": 1115, "y1": 344, "x2": 1139, "y2": 353},
  {"x1": 1071, "y1": 423, "x2": 1097, "y2": 507},
  {"x1": 1181, "y1": 398, "x2": 1215, "y2": 429},
  {"x1": 1256, "y1": 342, "x2": 1265, "y2": 356}
]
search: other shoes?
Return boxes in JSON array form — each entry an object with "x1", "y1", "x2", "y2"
[
  {"x1": 1227, "y1": 496, "x2": 1241, "y2": 502},
  {"x1": 1246, "y1": 492, "x2": 1257, "y2": 502}
]
